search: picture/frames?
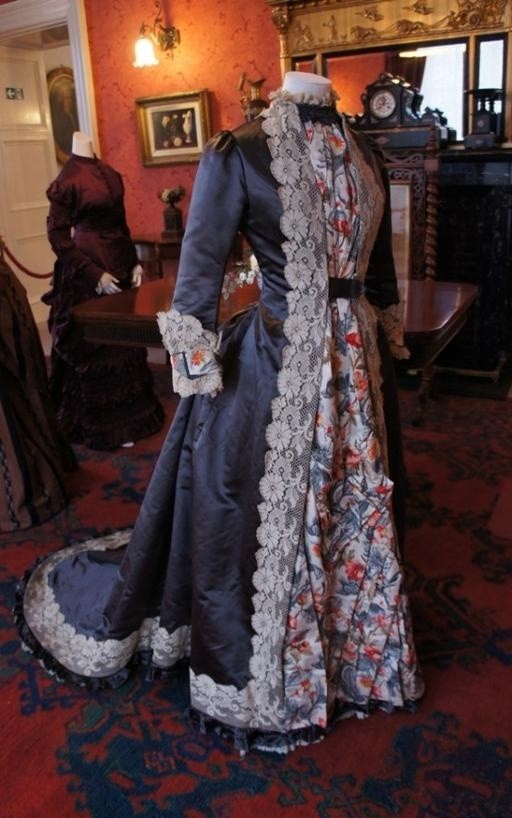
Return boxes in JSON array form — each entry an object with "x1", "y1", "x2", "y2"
[
  {"x1": 47, "y1": 68, "x2": 78, "y2": 163},
  {"x1": 136, "y1": 88, "x2": 213, "y2": 168}
]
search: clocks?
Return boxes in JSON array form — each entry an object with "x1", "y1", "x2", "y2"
[{"x1": 368, "y1": 89, "x2": 396, "y2": 119}]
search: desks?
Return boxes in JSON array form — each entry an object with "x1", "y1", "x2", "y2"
[{"x1": 68, "y1": 270, "x2": 478, "y2": 372}]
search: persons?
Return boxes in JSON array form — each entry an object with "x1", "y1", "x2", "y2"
[
  {"x1": 1, "y1": 260, "x2": 82, "y2": 533},
  {"x1": 156, "y1": 70, "x2": 412, "y2": 755},
  {"x1": 41, "y1": 132, "x2": 166, "y2": 449}
]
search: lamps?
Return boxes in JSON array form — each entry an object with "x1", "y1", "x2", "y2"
[{"x1": 131, "y1": 0, "x2": 181, "y2": 68}]
